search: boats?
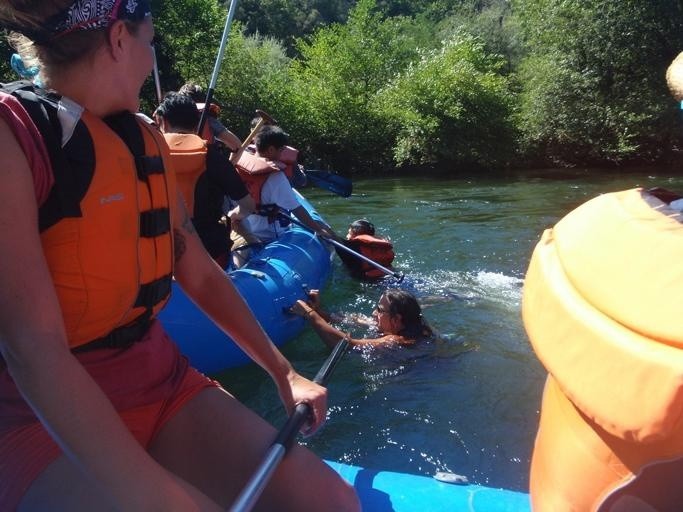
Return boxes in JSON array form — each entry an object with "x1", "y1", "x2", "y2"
[
  {"x1": 152, "y1": 186, "x2": 333, "y2": 378},
  {"x1": 318, "y1": 457, "x2": 528, "y2": 512}
]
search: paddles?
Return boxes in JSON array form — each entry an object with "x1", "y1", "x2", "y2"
[{"x1": 301, "y1": 170, "x2": 352, "y2": 198}]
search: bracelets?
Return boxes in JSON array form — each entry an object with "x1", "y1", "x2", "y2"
[{"x1": 304, "y1": 308, "x2": 315, "y2": 315}]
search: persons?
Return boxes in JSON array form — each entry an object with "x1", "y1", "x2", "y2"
[
  {"x1": 0, "y1": 0, "x2": 366, "y2": 512},
  {"x1": 288, "y1": 287, "x2": 439, "y2": 358},
  {"x1": 152, "y1": 80, "x2": 332, "y2": 270},
  {"x1": 314, "y1": 219, "x2": 396, "y2": 281},
  {"x1": 518, "y1": 50, "x2": 683, "y2": 512}
]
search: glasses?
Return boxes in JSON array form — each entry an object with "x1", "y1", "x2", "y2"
[{"x1": 377, "y1": 304, "x2": 391, "y2": 313}]
